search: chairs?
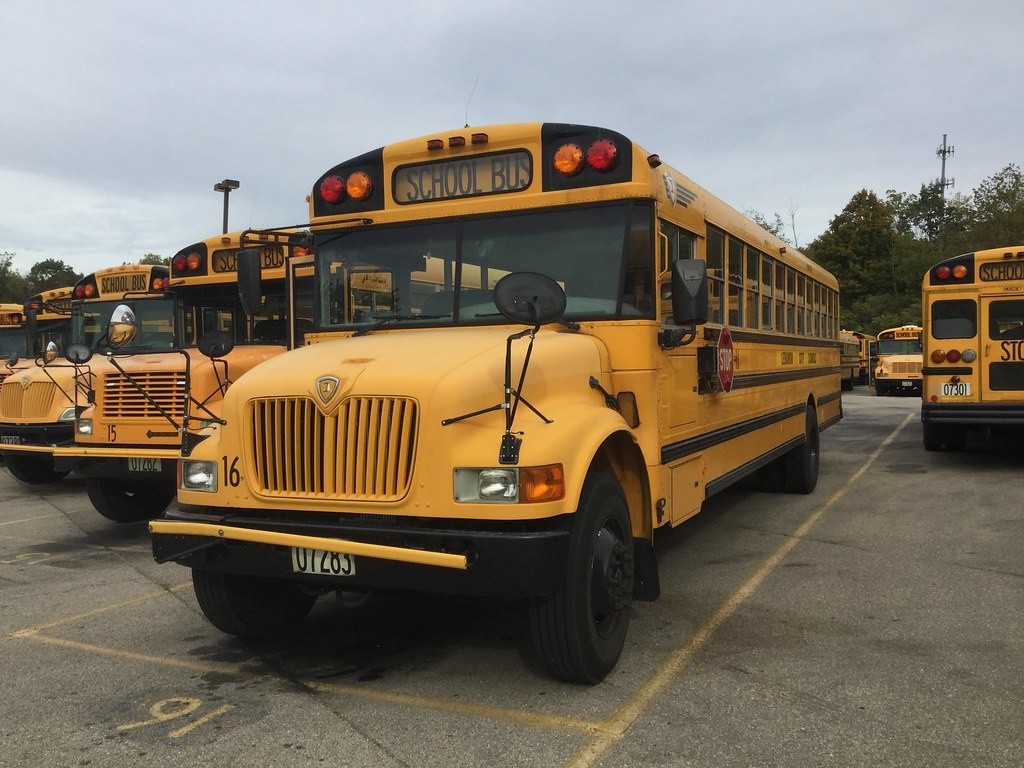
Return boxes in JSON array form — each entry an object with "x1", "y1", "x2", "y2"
[{"x1": 932, "y1": 318, "x2": 975, "y2": 339}]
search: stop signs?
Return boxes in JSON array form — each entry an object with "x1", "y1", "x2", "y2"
[{"x1": 715, "y1": 327, "x2": 739, "y2": 392}]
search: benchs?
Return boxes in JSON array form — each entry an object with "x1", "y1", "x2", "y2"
[
  {"x1": 420, "y1": 289, "x2": 494, "y2": 315},
  {"x1": 251, "y1": 319, "x2": 312, "y2": 340}
]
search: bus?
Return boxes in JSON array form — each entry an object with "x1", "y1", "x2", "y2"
[
  {"x1": 35, "y1": 225, "x2": 562, "y2": 522},
  {"x1": 839, "y1": 333, "x2": 860, "y2": 391},
  {"x1": 874, "y1": 325, "x2": 924, "y2": 395},
  {"x1": 840, "y1": 330, "x2": 878, "y2": 385},
  {"x1": 0, "y1": 303, "x2": 28, "y2": 383},
  {"x1": 0, "y1": 287, "x2": 232, "y2": 485},
  {"x1": 145, "y1": 121, "x2": 852, "y2": 682},
  {"x1": 920, "y1": 246, "x2": 1024, "y2": 451}
]
